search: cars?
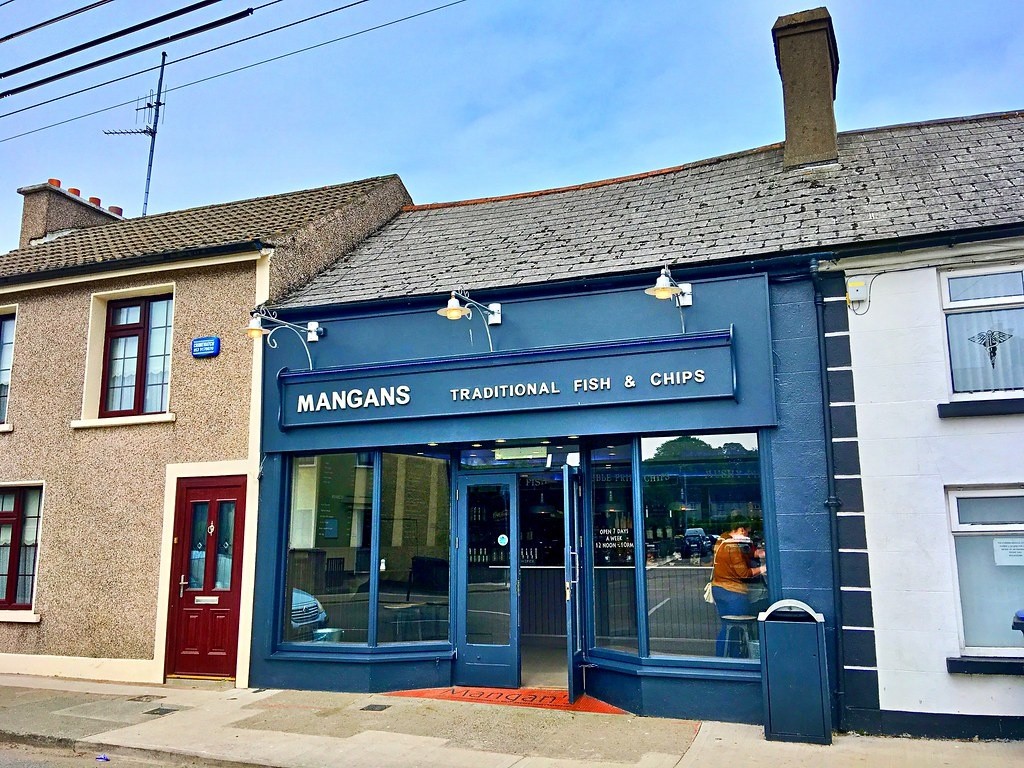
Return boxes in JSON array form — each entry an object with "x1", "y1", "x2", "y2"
[
  {"x1": 606, "y1": 549, "x2": 634, "y2": 564},
  {"x1": 706, "y1": 534, "x2": 721, "y2": 551},
  {"x1": 190, "y1": 552, "x2": 329, "y2": 641}
]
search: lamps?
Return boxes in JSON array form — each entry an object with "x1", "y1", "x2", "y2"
[
  {"x1": 435, "y1": 285, "x2": 502, "y2": 352},
  {"x1": 240, "y1": 303, "x2": 320, "y2": 371},
  {"x1": 644, "y1": 265, "x2": 693, "y2": 333}
]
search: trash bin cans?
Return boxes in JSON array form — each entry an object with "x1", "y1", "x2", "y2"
[
  {"x1": 757, "y1": 599, "x2": 832, "y2": 744},
  {"x1": 291, "y1": 548, "x2": 327, "y2": 594}
]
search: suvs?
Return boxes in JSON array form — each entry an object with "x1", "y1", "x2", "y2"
[{"x1": 684, "y1": 528, "x2": 707, "y2": 557}]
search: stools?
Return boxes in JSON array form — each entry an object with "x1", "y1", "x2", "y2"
[
  {"x1": 721, "y1": 615, "x2": 758, "y2": 656},
  {"x1": 384, "y1": 602, "x2": 428, "y2": 642}
]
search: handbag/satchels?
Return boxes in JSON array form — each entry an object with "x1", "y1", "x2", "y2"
[{"x1": 704, "y1": 583, "x2": 714, "y2": 603}]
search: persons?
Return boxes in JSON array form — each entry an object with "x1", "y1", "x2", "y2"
[{"x1": 709, "y1": 514, "x2": 767, "y2": 660}]
